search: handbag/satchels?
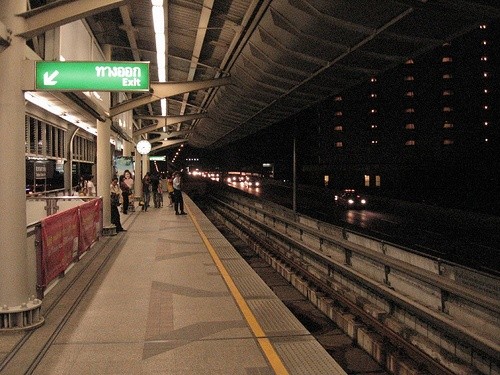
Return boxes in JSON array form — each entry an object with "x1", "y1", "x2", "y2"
[
  {"x1": 171, "y1": 192, "x2": 175, "y2": 203},
  {"x1": 139, "y1": 200, "x2": 145, "y2": 206},
  {"x1": 129, "y1": 189, "x2": 135, "y2": 194}
]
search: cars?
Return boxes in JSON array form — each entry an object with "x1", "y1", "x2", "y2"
[
  {"x1": 334, "y1": 189, "x2": 367, "y2": 209},
  {"x1": 189, "y1": 168, "x2": 262, "y2": 189}
]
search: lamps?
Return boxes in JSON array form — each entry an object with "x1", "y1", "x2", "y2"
[{"x1": 151, "y1": 0, "x2": 169, "y2": 115}]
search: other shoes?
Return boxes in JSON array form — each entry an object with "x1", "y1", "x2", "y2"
[
  {"x1": 154, "y1": 205, "x2": 163, "y2": 208},
  {"x1": 121, "y1": 229, "x2": 127, "y2": 232},
  {"x1": 181, "y1": 212, "x2": 187, "y2": 215},
  {"x1": 176, "y1": 212, "x2": 179, "y2": 215}
]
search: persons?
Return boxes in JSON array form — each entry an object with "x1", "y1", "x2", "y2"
[
  {"x1": 119, "y1": 175, "x2": 131, "y2": 215},
  {"x1": 78, "y1": 169, "x2": 173, "y2": 231},
  {"x1": 172, "y1": 172, "x2": 188, "y2": 215}
]
